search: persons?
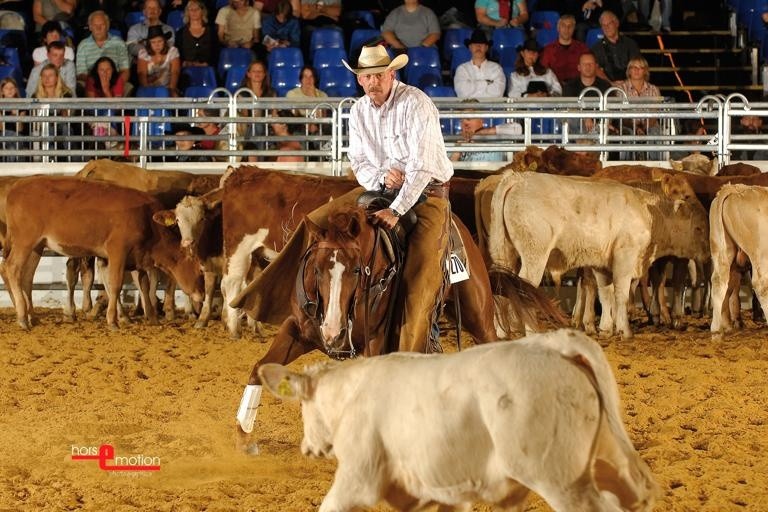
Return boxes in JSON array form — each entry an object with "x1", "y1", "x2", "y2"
[
  {"x1": 339, "y1": 39, "x2": 455, "y2": 353},
  {"x1": 2, "y1": 1, "x2": 767, "y2": 161}
]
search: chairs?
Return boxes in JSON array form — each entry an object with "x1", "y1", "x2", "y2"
[
  {"x1": 0, "y1": 0, "x2": 321, "y2": 144},
  {"x1": 321, "y1": 0, "x2": 633, "y2": 149}
]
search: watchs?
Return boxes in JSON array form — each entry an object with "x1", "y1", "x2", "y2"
[{"x1": 391, "y1": 208, "x2": 400, "y2": 217}]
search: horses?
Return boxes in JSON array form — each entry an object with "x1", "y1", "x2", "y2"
[{"x1": 233, "y1": 200, "x2": 576, "y2": 444}]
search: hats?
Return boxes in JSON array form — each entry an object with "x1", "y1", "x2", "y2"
[
  {"x1": 164, "y1": 123, "x2": 205, "y2": 144},
  {"x1": 143, "y1": 25, "x2": 172, "y2": 41},
  {"x1": 463, "y1": 30, "x2": 493, "y2": 48},
  {"x1": 516, "y1": 40, "x2": 543, "y2": 51},
  {"x1": 342, "y1": 45, "x2": 409, "y2": 75}
]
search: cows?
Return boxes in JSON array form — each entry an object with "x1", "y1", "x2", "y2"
[
  {"x1": 0, "y1": 144, "x2": 768, "y2": 340},
  {"x1": 256, "y1": 326, "x2": 663, "y2": 512}
]
